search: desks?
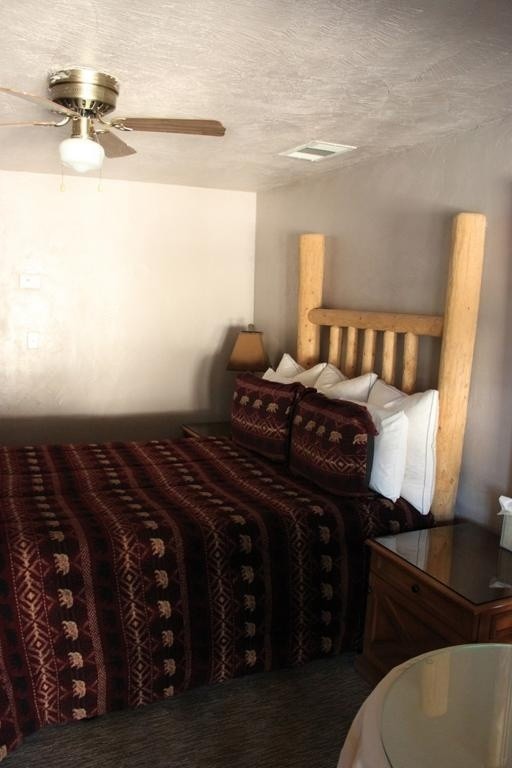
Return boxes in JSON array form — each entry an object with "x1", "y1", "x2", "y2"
[{"x1": 336, "y1": 643, "x2": 512, "y2": 768}]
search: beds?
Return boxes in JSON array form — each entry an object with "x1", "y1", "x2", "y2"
[{"x1": 0, "y1": 212, "x2": 488, "y2": 762}]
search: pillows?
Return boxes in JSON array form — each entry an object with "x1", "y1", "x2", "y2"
[
  {"x1": 368, "y1": 380, "x2": 439, "y2": 516},
  {"x1": 314, "y1": 364, "x2": 378, "y2": 402},
  {"x1": 289, "y1": 386, "x2": 379, "y2": 497},
  {"x1": 261, "y1": 363, "x2": 327, "y2": 388},
  {"x1": 229, "y1": 371, "x2": 304, "y2": 466},
  {"x1": 275, "y1": 353, "x2": 306, "y2": 378},
  {"x1": 341, "y1": 398, "x2": 408, "y2": 503}
]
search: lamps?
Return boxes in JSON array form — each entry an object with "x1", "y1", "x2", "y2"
[
  {"x1": 226, "y1": 331, "x2": 270, "y2": 375},
  {"x1": 58, "y1": 117, "x2": 105, "y2": 172}
]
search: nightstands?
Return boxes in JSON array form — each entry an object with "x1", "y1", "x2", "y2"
[
  {"x1": 180, "y1": 421, "x2": 230, "y2": 438},
  {"x1": 363, "y1": 522, "x2": 512, "y2": 673}
]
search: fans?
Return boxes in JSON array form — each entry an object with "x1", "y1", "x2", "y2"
[{"x1": 0, "y1": 68, "x2": 226, "y2": 159}]
